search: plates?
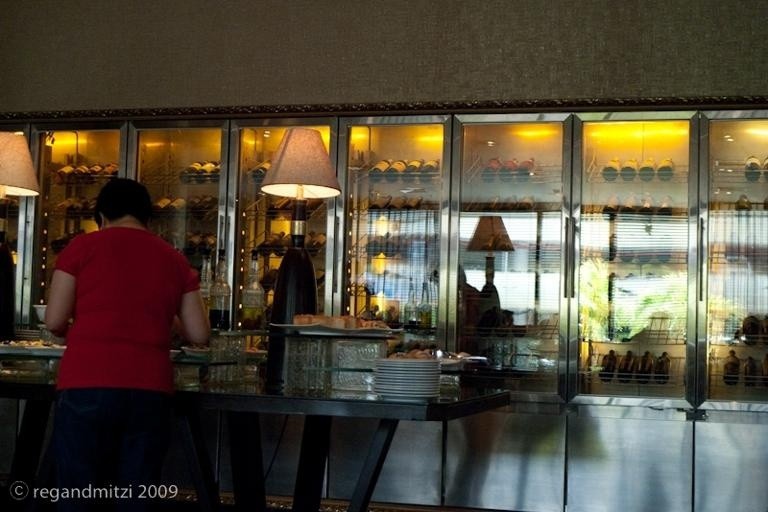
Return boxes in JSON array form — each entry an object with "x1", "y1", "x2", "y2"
[
  {"x1": 0, "y1": 341, "x2": 68, "y2": 359},
  {"x1": 372, "y1": 355, "x2": 441, "y2": 406}
]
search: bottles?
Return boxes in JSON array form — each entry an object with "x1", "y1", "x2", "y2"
[{"x1": 47, "y1": 155, "x2": 766, "y2": 384}]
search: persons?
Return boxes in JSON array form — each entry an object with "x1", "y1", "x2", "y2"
[
  {"x1": 427, "y1": 260, "x2": 481, "y2": 354},
  {"x1": 42, "y1": 176, "x2": 212, "y2": 512},
  {"x1": 0, "y1": 238, "x2": 17, "y2": 368},
  {"x1": 501, "y1": 308, "x2": 514, "y2": 335}
]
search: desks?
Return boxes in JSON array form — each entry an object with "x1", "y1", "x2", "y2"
[{"x1": 1, "y1": 361, "x2": 509, "y2": 512}]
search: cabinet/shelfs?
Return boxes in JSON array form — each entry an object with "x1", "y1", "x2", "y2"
[
  {"x1": 446, "y1": 111, "x2": 699, "y2": 410},
  {"x1": 225, "y1": 114, "x2": 451, "y2": 353},
  {"x1": 22, "y1": 113, "x2": 231, "y2": 333},
  {"x1": 699, "y1": 108, "x2": 768, "y2": 410},
  {"x1": 0, "y1": 121, "x2": 31, "y2": 327}
]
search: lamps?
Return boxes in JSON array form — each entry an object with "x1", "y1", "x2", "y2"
[
  {"x1": 0, "y1": 131, "x2": 40, "y2": 339},
  {"x1": 467, "y1": 217, "x2": 514, "y2": 308},
  {"x1": 259, "y1": 126, "x2": 339, "y2": 383}
]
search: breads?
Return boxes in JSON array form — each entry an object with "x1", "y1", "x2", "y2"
[
  {"x1": 292, "y1": 312, "x2": 392, "y2": 330},
  {"x1": 390, "y1": 348, "x2": 436, "y2": 360}
]
